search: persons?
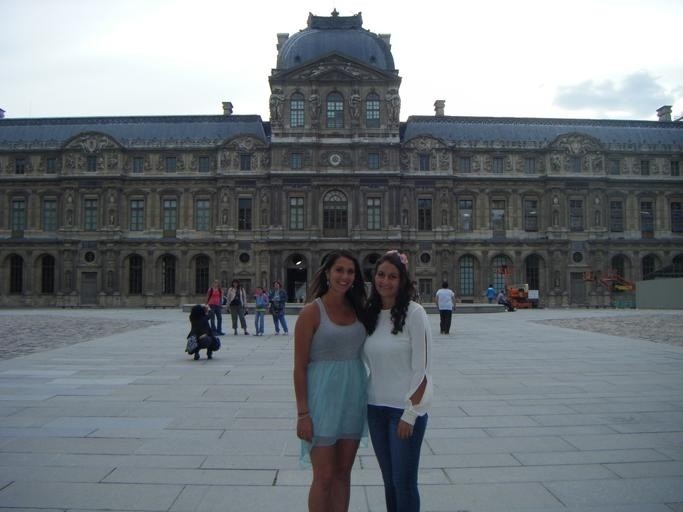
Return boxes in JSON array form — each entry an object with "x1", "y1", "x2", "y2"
[
  {"x1": 293, "y1": 249, "x2": 369, "y2": 512},
  {"x1": 254, "y1": 287, "x2": 266, "y2": 334},
  {"x1": 269, "y1": 281, "x2": 288, "y2": 335},
  {"x1": 203, "y1": 279, "x2": 224, "y2": 336},
  {"x1": 186, "y1": 305, "x2": 214, "y2": 362},
  {"x1": 497, "y1": 290, "x2": 516, "y2": 311},
  {"x1": 435, "y1": 282, "x2": 455, "y2": 334},
  {"x1": 358, "y1": 250, "x2": 435, "y2": 512},
  {"x1": 225, "y1": 279, "x2": 249, "y2": 335},
  {"x1": 486, "y1": 284, "x2": 495, "y2": 304}
]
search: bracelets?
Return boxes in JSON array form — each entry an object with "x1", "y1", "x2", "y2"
[
  {"x1": 297, "y1": 410, "x2": 308, "y2": 415},
  {"x1": 297, "y1": 414, "x2": 308, "y2": 420}
]
